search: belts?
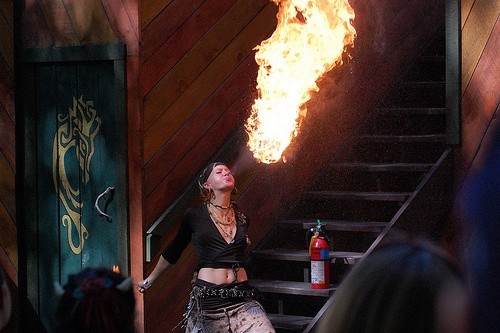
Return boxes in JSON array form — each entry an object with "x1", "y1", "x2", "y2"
[{"x1": 172, "y1": 285, "x2": 256, "y2": 333}]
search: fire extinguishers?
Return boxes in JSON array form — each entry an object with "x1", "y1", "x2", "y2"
[{"x1": 306, "y1": 219, "x2": 332, "y2": 290}]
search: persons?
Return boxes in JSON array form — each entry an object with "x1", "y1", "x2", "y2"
[
  {"x1": 54, "y1": 266, "x2": 136, "y2": 333},
  {"x1": 142, "y1": 161, "x2": 276, "y2": 333},
  {"x1": 316, "y1": 241, "x2": 470, "y2": 333}
]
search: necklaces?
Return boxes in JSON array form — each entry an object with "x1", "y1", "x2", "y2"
[{"x1": 208, "y1": 201, "x2": 236, "y2": 238}]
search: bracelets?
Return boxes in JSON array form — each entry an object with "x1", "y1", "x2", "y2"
[{"x1": 143, "y1": 278, "x2": 152, "y2": 288}]
